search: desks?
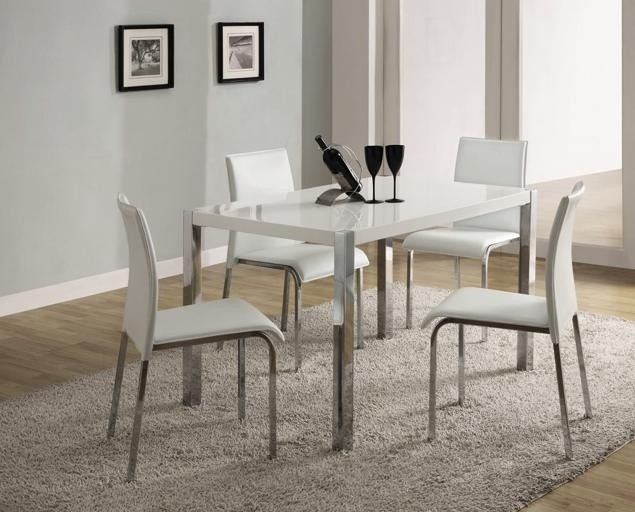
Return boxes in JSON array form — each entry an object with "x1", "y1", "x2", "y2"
[{"x1": 180, "y1": 175, "x2": 538, "y2": 452}]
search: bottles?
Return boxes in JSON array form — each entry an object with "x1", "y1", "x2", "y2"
[{"x1": 315, "y1": 135, "x2": 363, "y2": 201}]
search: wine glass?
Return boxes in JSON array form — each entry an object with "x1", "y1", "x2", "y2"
[{"x1": 364, "y1": 145, "x2": 404, "y2": 204}]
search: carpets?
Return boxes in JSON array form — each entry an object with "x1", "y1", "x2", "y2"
[{"x1": 0, "y1": 278, "x2": 635, "y2": 512}]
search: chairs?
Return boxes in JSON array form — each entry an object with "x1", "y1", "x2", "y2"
[
  {"x1": 399, "y1": 133, "x2": 530, "y2": 343},
  {"x1": 106, "y1": 191, "x2": 285, "y2": 483},
  {"x1": 419, "y1": 179, "x2": 592, "y2": 461},
  {"x1": 216, "y1": 147, "x2": 369, "y2": 368}
]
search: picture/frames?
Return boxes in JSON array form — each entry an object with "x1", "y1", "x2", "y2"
[{"x1": 115, "y1": 21, "x2": 265, "y2": 93}]
